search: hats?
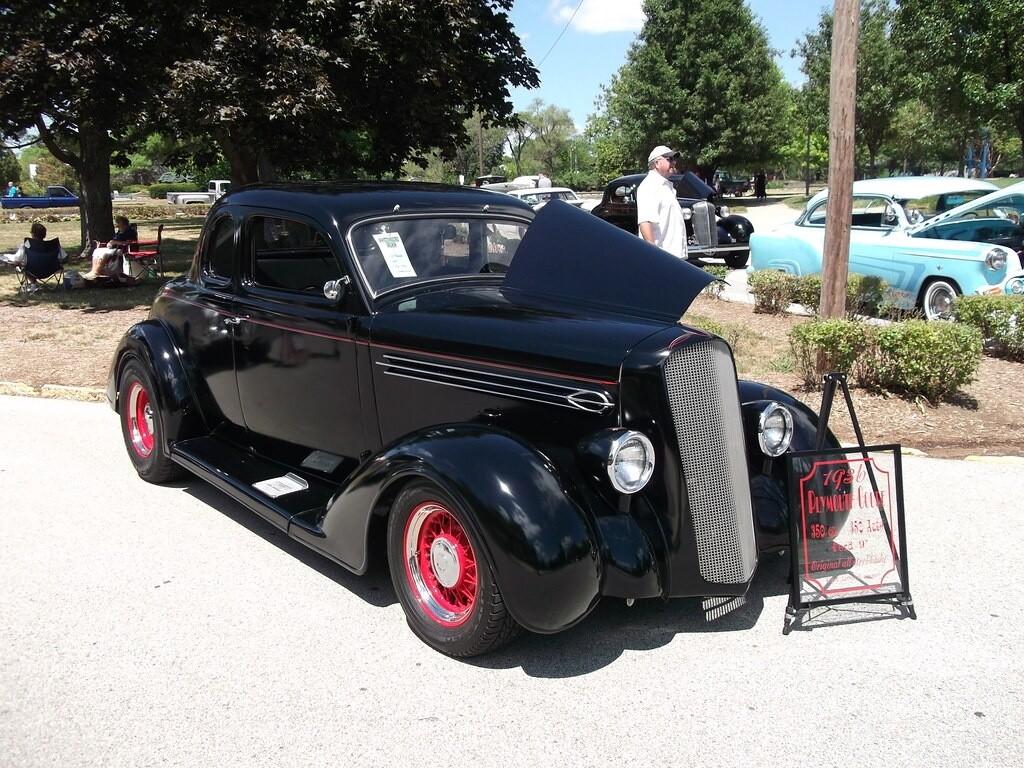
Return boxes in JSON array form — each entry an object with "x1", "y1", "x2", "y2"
[
  {"x1": 647, "y1": 146, "x2": 681, "y2": 159},
  {"x1": 18, "y1": 186, "x2": 22, "y2": 191}
]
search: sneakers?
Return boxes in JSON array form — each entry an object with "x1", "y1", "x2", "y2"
[
  {"x1": 22, "y1": 285, "x2": 38, "y2": 292},
  {"x1": 81, "y1": 271, "x2": 97, "y2": 280}
]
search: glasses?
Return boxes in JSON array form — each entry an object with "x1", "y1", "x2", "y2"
[{"x1": 30, "y1": 231, "x2": 31, "y2": 233}]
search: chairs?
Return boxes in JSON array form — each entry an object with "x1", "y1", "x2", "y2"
[
  {"x1": 851, "y1": 213, "x2": 945, "y2": 229},
  {"x1": 94, "y1": 223, "x2": 166, "y2": 283},
  {"x1": 251, "y1": 253, "x2": 501, "y2": 312},
  {"x1": 14, "y1": 237, "x2": 66, "y2": 292}
]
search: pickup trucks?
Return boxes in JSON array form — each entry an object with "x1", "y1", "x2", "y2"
[
  {"x1": 0, "y1": 186, "x2": 81, "y2": 209},
  {"x1": 167, "y1": 180, "x2": 232, "y2": 205}
]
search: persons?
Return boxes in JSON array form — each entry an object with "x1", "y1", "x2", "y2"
[
  {"x1": 637, "y1": 145, "x2": 688, "y2": 260},
  {"x1": 5, "y1": 182, "x2": 23, "y2": 197},
  {"x1": 755, "y1": 170, "x2": 770, "y2": 202},
  {"x1": 537, "y1": 174, "x2": 551, "y2": 188},
  {"x1": 79, "y1": 216, "x2": 138, "y2": 280},
  {"x1": 7, "y1": 224, "x2": 67, "y2": 292}
]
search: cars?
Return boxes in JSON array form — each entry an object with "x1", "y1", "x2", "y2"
[
  {"x1": 591, "y1": 168, "x2": 754, "y2": 270},
  {"x1": 505, "y1": 187, "x2": 592, "y2": 216},
  {"x1": 747, "y1": 170, "x2": 1024, "y2": 324},
  {"x1": 105, "y1": 179, "x2": 857, "y2": 664}
]
